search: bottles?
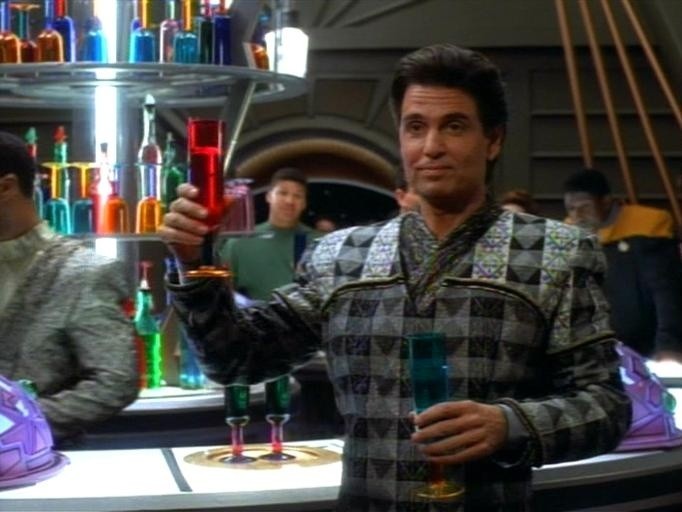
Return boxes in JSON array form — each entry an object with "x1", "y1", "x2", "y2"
[
  {"x1": 1, "y1": 0, "x2": 233, "y2": 66},
  {"x1": 27, "y1": 89, "x2": 180, "y2": 221},
  {"x1": 129, "y1": 253, "x2": 204, "y2": 393}
]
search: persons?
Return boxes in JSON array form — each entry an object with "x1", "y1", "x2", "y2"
[
  {"x1": 503, "y1": 169, "x2": 681, "y2": 366},
  {"x1": 155, "y1": 44, "x2": 632, "y2": 511},
  {"x1": 0, "y1": 130, "x2": 142, "y2": 450},
  {"x1": 393, "y1": 171, "x2": 421, "y2": 215},
  {"x1": 217, "y1": 167, "x2": 340, "y2": 305}
]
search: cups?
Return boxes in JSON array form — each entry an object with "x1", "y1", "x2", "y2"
[{"x1": 184, "y1": 116, "x2": 236, "y2": 282}]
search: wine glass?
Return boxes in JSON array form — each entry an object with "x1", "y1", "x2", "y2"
[
  {"x1": 37, "y1": 160, "x2": 162, "y2": 233},
  {"x1": 225, "y1": 374, "x2": 295, "y2": 463},
  {"x1": 406, "y1": 332, "x2": 469, "y2": 500}
]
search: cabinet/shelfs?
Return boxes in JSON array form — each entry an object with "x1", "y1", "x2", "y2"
[{"x1": 0, "y1": 1, "x2": 302, "y2": 448}]
